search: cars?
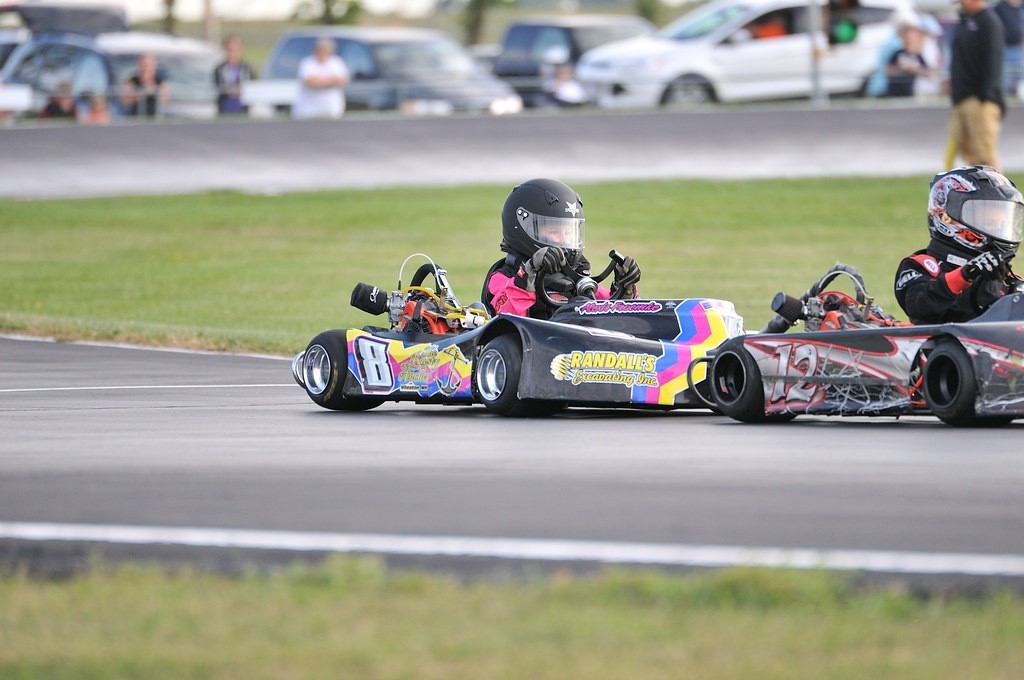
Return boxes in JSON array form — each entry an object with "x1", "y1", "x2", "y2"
[
  {"x1": 493, "y1": 18, "x2": 656, "y2": 111},
  {"x1": 263, "y1": 27, "x2": 525, "y2": 118},
  {"x1": 576, "y1": 0, "x2": 940, "y2": 110},
  {"x1": 0, "y1": 34, "x2": 277, "y2": 120}
]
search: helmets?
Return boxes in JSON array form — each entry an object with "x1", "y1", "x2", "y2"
[
  {"x1": 500, "y1": 179, "x2": 585, "y2": 269},
  {"x1": 927, "y1": 165, "x2": 1024, "y2": 264}
]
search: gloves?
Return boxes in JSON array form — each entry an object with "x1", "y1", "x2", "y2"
[
  {"x1": 962, "y1": 251, "x2": 1006, "y2": 284},
  {"x1": 524, "y1": 247, "x2": 567, "y2": 276},
  {"x1": 613, "y1": 256, "x2": 642, "y2": 294}
]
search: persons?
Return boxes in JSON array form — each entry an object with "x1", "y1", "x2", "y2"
[
  {"x1": 291, "y1": 36, "x2": 351, "y2": 117},
  {"x1": 893, "y1": 165, "x2": 1024, "y2": 325},
  {"x1": 87, "y1": 96, "x2": 110, "y2": 123},
  {"x1": 37, "y1": 82, "x2": 78, "y2": 119},
  {"x1": 480, "y1": 178, "x2": 641, "y2": 321},
  {"x1": 745, "y1": 13, "x2": 789, "y2": 37},
  {"x1": 867, "y1": 22, "x2": 941, "y2": 97},
  {"x1": 215, "y1": 33, "x2": 258, "y2": 112},
  {"x1": 948, "y1": 0, "x2": 1005, "y2": 167},
  {"x1": 120, "y1": 55, "x2": 170, "y2": 116},
  {"x1": 992, "y1": 0, "x2": 1024, "y2": 87}
]
